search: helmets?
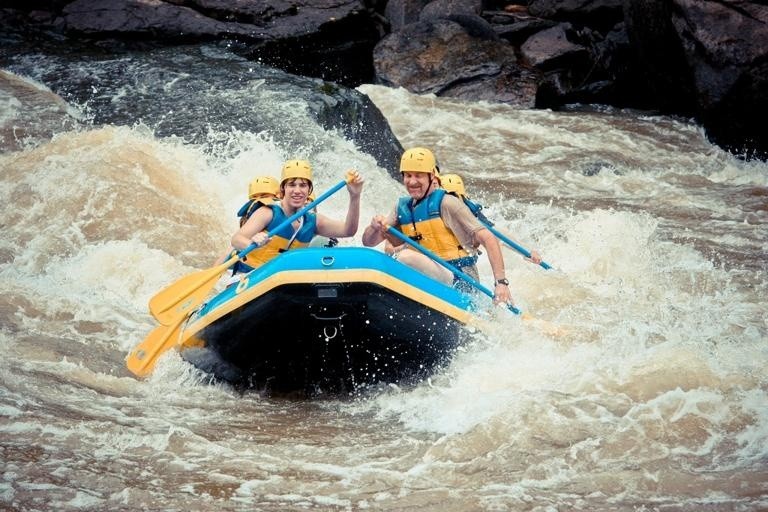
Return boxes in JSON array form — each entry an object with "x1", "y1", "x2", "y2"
[
  {"x1": 433, "y1": 166, "x2": 442, "y2": 186},
  {"x1": 398, "y1": 146, "x2": 436, "y2": 174},
  {"x1": 306, "y1": 192, "x2": 316, "y2": 202},
  {"x1": 279, "y1": 159, "x2": 314, "y2": 192},
  {"x1": 248, "y1": 175, "x2": 281, "y2": 201},
  {"x1": 439, "y1": 173, "x2": 466, "y2": 196}
]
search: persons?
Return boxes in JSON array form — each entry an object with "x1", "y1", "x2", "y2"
[
  {"x1": 215, "y1": 158, "x2": 363, "y2": 286},
  {"x1": 363, "y1": 147, "x2": 515, "y2": 306},
  {"x1": 386, "y1": 168, "x2": 540, "y2": 263}
]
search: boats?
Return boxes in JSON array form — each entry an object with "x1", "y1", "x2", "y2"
[{"x1": 179, "y1": 246, "x2": 495, "y2": 395}]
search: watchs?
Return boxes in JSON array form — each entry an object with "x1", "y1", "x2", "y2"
[{"x1": 495, "y1": 278, "x2": 509, "y2": 287}]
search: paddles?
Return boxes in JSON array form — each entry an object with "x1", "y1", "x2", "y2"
[
  {"x1": 127, "y1": 249, "x2": 238, "y2": 378},
  {"x1": 149, "y1": 173, "x2": 354, "y2": 327}
]
